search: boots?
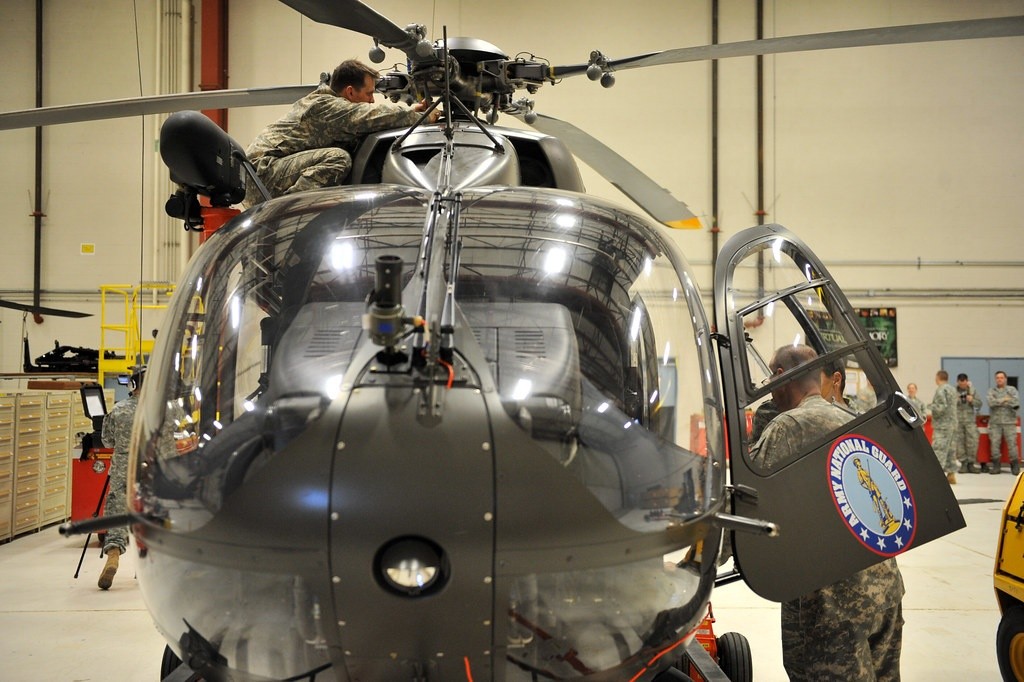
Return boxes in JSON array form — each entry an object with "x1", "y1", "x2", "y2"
[
  {"x1": 989, "y1": 459, "x2": 1001, "y2": 474},
  {"x1": 98, "y1": 547, "x2": 120, "y2": 588},
  {"x1": 1010, "y1": 459, "x2": 1020, "y2": 475},
  {"x1": 958, "y1": 460, "x2": 969, "y2": 472},
  {"x1": 968, "y1": 463, "x2": 980, "y2": 473},
  {"x1": 947, "y1": 473, "x2": 956, "y2": 484}
]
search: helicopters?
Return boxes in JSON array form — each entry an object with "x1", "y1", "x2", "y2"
[{"x1": 0, "y1": 0, "x2": 1024, "y2": 682}]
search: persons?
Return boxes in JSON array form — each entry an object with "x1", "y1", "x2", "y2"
[
  {"x1": 987, "y1": 371, "x2": 1021, "y2": 476},
  {"x1": 98, "y1": 369, "x2": 184, "y2": 590},
  {"x1": 723, "y1": 344, "x2": 905, "y2": 682},
  {"x1": 907, "y1": 383, "x2": 927, "y2": 432},
  {"x1": 240, "y1": 60, "x2": 441, "y2": 307},
  {"x1": 955, "y1": 373, "x2": 984, "y2": 473},
  {"x1": 858, "y1": 378, "x2": 877, "y2": 412},
  {"x1": 927, "y1": 371, "x2": 959, "y2": 484}
]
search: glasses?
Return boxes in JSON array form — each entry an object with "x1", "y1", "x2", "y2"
[{"x1": 761, "y1": 371, "x2": 778, "y2": 385}]
